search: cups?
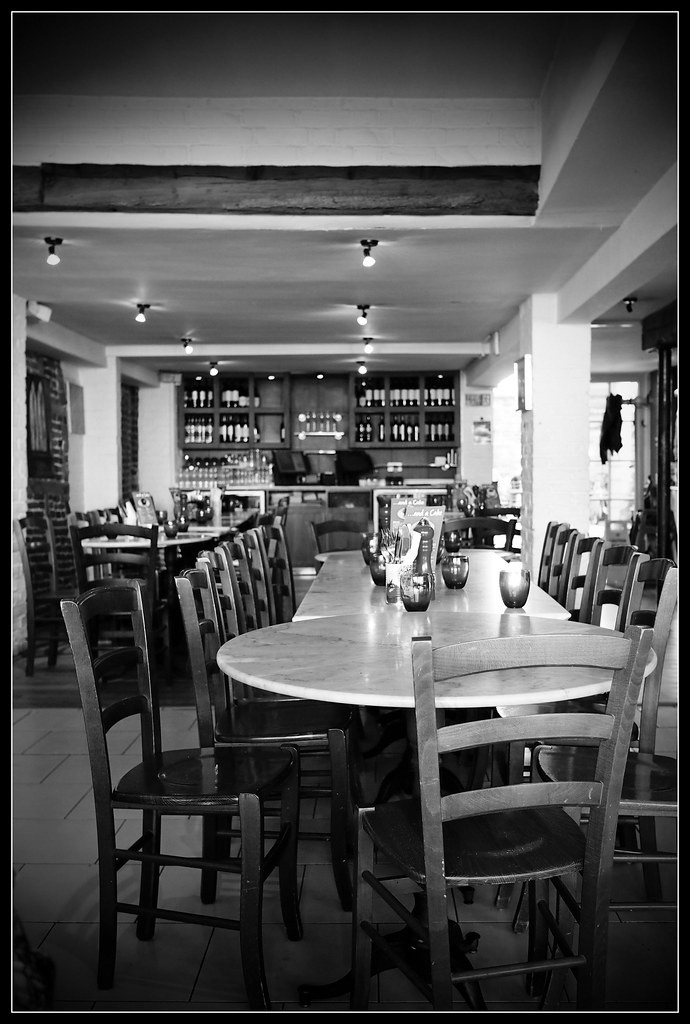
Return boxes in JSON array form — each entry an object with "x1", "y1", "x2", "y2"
[
  {"x1": 369, "y1": 553, "x2": 386, "y2": 585},
  {"x1": 195, "y1": 510, "x2": 208, "y2": 524},
  {"x1": 361, "y1": 532, "x2": 382, "y2": 565},
  {"x1": 204, "y1": 507, "x2": 214, "y2": 521},
  {"x1": 400, "y1": 573, "x2": 432, "y2": 611},
  {"x1": 385, "y1": 563, "x2": 402, "y2": 604},
  {"x1": 104, "y1": 521, "x2": 119, "y2": 540},
  {"x1": 500, "y1": 569, "x2": 531, "y2": 608},
  {"x1": 444, "y1": 531, "x2": 462, "y2": 553},
  {"x1": 441, "y1": 556, "x2": 470, "y2": 590},
  {"x1": 157, "y1": 510, "x2": 167, "y2": 526},
  {"x1": 163, "y1": 520, "x2": 178, "y2": 538}
]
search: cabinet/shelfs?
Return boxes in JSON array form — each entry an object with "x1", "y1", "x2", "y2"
[{"x1": 170, "y1": 371, "x2": 462, "y2": 505}]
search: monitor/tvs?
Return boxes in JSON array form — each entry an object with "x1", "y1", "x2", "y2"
[{"x1": 273, "y1": 449, "x2": 307, "y2": 474}]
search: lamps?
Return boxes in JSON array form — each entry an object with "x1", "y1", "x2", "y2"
[
  {"x1": 623, "y1": 297, "x2": 638, "y2": 313},
  {"x1": 363, "y1": 338, "x2": 374, "y2": 353},
  {"x1": 180, "y1": 339, "x2": 194, "y2": 354},
  {"x1": 136, "y1": 304, "x2": 150, "y2": 322},
  {"x1": 210, "y1": 362, "x2": 219, "y2": 376},
  {"x1": 361, "y1": 240, "x2": 379, "y2": 266},
  {"x1": 44, "y1": 238, "x2": 63, "y2": 264},
  {"x1": 356, "y1": 305, "x2": 370, "y2": 325},
  {"x1": 358, "y1": 361, "x2": 368, "y2": 374}
]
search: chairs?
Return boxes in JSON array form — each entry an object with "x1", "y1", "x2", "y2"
[{"x1": 13, "y1": 492, "x2": 680, "y2": 1012}]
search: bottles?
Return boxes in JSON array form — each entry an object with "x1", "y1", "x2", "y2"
[
  {"x1": 279, "y1": 420, "x2": 285, "y2": 443},
  {"x1": 413, "y1": 526, "x2": 435, "y2": 599},
  {"x1": 176, "y1": 494, "x2": 190, "y2": 531},
  {"x1": 387, "y1": 463, "x2": 403, "y2": 488},
  {"x1": 182, "y1": 379, "x2": 273, "y2": 489},
  {"x1": 356, "y1": 381, "x2": 455, "y2": 442}
]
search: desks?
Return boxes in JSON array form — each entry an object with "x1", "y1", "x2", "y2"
[
  {"x1": 214, "y1": 609, "x2": 664, "y2": 1014},
  {"x1": 291, "y1": 546, "x2": 574, "y2": 804},
  {"x1": 79, "y1": 536, "x2": 210, "y2": 683},
  {"x1": 182, "y1": 507, "x2": 261, "y2": 533}
]
